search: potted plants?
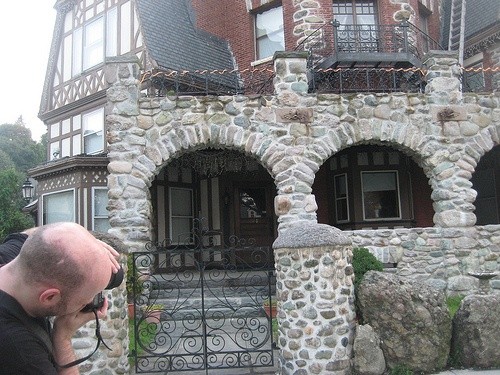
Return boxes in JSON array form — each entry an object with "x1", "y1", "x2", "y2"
[
  {"x1": 270, "y1": 319, "x2": 278, "y2": 344},
  {"x1": 141, "y1": 303, "x2": 165, "y2": 324},
  {"x1": 262, "y1": 297, "x2": 277, "y2": 317}
]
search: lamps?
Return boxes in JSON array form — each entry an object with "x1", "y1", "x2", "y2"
[{"x1": 21, "y1": 177, "x2": 34, "y2": 203}]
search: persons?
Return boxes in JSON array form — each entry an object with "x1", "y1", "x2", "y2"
[{"x1": 1, "y1": 221, "x2": 120, "y2": 375}]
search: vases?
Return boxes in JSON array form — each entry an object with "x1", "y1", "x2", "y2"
[{"x1": 128, "y1": 304, "x2": 135, "y2": 320}]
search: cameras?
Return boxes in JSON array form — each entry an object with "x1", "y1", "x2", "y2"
[{"x1": 82, "y1": 264, "x2": 124, "y2": 312}]
